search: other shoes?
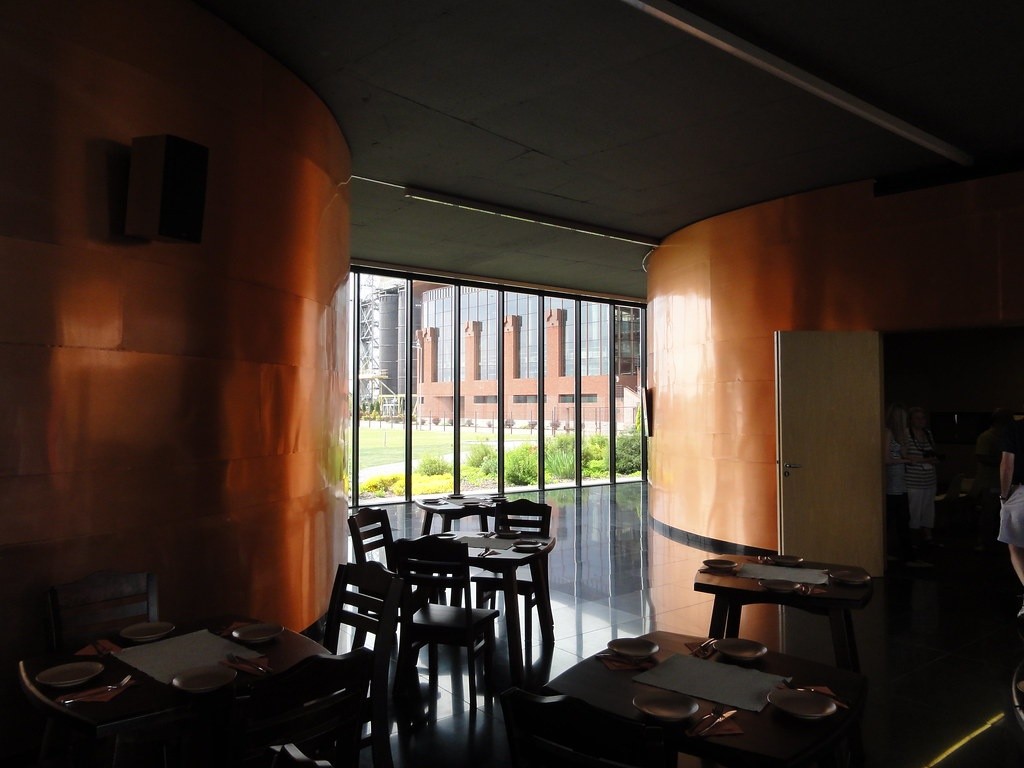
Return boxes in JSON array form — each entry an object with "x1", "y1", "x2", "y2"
[
  {"x1": 1017, "y1": 595, "x2": 1024, "y2": 620},
  {"x1": 912, "y1": 542, "x2": 921, "y2": 550},
  {"x1": 926, "y1": 538, "x2": 945, "y2": 548}
]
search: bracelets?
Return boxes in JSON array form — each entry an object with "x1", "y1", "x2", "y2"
[{"x1": 1000, "y1": 496, "x2": 1007, "y2": 501}]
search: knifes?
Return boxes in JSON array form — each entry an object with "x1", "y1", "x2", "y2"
[
  {"x1": 236, "y1": 655, "x2": 273, "y2": 672},
  {"x1": 690, "y1": 638, "x2": 714, "y2": 654},
  {"x1": 700, "y1": 710, "x2": 738, "y2": 734}
]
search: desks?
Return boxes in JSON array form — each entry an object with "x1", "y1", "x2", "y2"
[
  {"x1": 542, "y1": 629, "x2": 855, "y2": 768},
  {"x1": 694, "y1": 554, "x2": 873, "y2": 672},
  {"x1": 421, "y1": 534, "x2": 557, "y2": 670},
  {"x1": 17, "y1": 615, "x2": 334, "y2": 768}
]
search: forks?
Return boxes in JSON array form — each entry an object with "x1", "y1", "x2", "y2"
[
  {"x1": 482, "y1": 547, "x2": 489, "y2": 556},
  {"x1": 782, "y1": 679, "x2": 851, "y2": 709},
  {"x1": 63, "y1": 675, "x2": 131, "y2": 704},
  {"x1": 226, "y1": 652, "x2": 266, "y2": 672},
  {"x1": 688, "y1": 704, "x2": 723, "y2": 734}
]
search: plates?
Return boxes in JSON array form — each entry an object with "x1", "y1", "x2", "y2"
[
  {"x1": 423, "y1": 501, "x2": 439, "y2": 503},
  {"x1": 439, "y1": 536, "x2": 456, "y2": 540},
  {"x1": 772, "y1": 555, "x2": 804, "y2": 564},
  {"x1": 633, "y1": 691, "x2": 700, "y2": 721},
  {"x1": 766, "y1": 689, "x2": 837, "y2": 720},
  {"x1": 515, "y1": 544, "x2": 541, "y2": 549},
  {"x1": 463, "y1": 504, "x2": 479, "y2": 507},
  {"x1": 120, "y1": 621, "x2": 176, "y2": 642},
  {"x1": 491, "y1": 497, "x2": 507, "y2": 501},
  {"x1": 713, "y1": 638, "x2": 768, "y2": 661},
  {"x1": 607, "y1": 638, "x2": 660, "y2": 658},
  {"x1": 758, "y1": 579, "x2": 801, "y2": 593},
  {"x1": 830, "y1": 571, "x2": 871, "y2": 584},
  {"x1": 232, "y1": 623, "x2": 285, "y2": 643},
  {"x1": 173, "y1": 666, "x2": 237, "y2": 693},
  {"x1": 703, "y1": 560, "x2": 738, "y2": 568},
  {"x1": 35, "y1": 661, "x2": 104, "y2": 687},
  {"x1": 449, "y1": 495, "x2": 464, "y2": 498},
  {"x1": 498, "y1": 532, "x2": 521, "y2": 535}
]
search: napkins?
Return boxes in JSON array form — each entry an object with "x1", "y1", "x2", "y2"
[
  {"x1": 219, "y1": 659, "x2": 269, "y2": 677},
  {"x1": 599, "y1": 657, "x2": 654, "y2": 672},
  {"x1": 74, "y1": 638, "x2": 122, "y2": 656},
  {"x1": 686, "y1": 713, "x2": 744, "y2": 737},
  {"x1": 794, "y1": 583, "x2": 827, "y2": 598},
  {"x1": 54, "y1": 679, "x2": 134, "y2": 705},
  {"x1": 219, "y1": 620, "x2": 250, "y2": 638},
  {"x1": 777, "y1": 683, "x2": 836, "y2": 698},
  {"x1": 684, "y1": 642, "x2": 717, "y2": 660}
]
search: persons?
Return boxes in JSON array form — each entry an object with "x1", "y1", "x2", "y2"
[
  {"x1": 883, "y1": 402, "x2": 939, "y2": 560},
  {"x1": 997, "y1": 401, "x2": 1024, "y2": 617}
]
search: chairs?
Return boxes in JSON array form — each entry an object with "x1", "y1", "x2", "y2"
[{"x1": 37, "y1": 490, "x2": 700, "y2": 768}]
